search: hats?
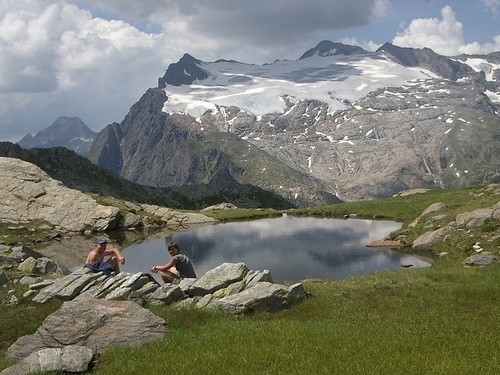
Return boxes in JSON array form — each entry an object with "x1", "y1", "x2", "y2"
[{"x1": 95, "y1": 237, "x2": 108, "y2": 246}]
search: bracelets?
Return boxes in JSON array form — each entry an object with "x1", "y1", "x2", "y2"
[{"x1": 154, "y1": 266, "x2": 156, "y2": 269}]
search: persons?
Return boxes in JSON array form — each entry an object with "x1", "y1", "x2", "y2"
[
  {"x1": 149, "y1": 241, "x2": 196, "y2": 285},
  {"x1": 84, "y1": 237, "x2": 126, "y2": 276}
]
